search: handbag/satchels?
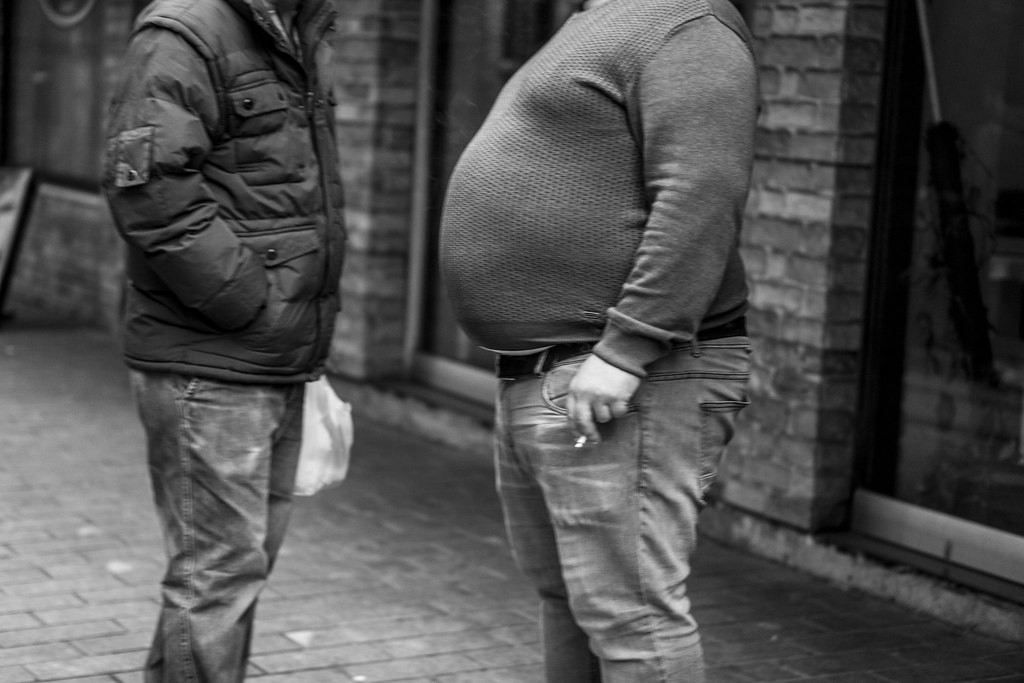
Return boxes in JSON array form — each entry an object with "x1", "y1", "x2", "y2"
[{"x1": 292, "y1": 374, "x2": 357, "y2": 497}]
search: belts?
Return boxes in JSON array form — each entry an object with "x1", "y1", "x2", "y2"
[{"x1": 495, "y1": 314, "x2": 748, "y2": 382}]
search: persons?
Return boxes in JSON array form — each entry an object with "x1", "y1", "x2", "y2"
[
  {"x1": 108, "y1": 0, "x2": 349, "y2": 683},
  {"x1": 442, "y1": 1, "x2": 760, "y2": 683}
]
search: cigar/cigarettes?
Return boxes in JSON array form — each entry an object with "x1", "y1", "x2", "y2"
[{"x1": 574, "y1": 435, "x2": 587, "y2": 448}]
